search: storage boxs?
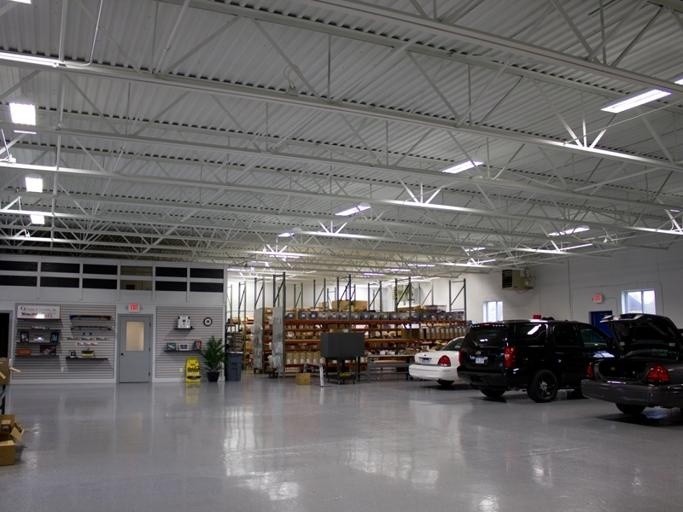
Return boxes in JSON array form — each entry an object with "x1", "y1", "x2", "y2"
[
  {"x1": 0, "y1": 357, "x2": 22, "y2": 385},
  {"x1": 0, "y1": 414, "x2": 24, "y2": 465}
]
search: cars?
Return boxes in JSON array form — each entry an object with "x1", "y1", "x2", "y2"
[
  {"x1": 408, "y1": 336, "x2": 478, "y2": 389},
  {"x1": 581, "y1": 311, "x2": 682, "y2": 416}
]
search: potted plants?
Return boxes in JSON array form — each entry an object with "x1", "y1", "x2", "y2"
[{"x1": 195, "y1": 335, "x2": 232, "y2": 382}]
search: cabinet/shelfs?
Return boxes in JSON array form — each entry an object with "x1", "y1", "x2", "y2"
[{"x1": 227, "y1": 308, "x2": 466, "y2": 379}]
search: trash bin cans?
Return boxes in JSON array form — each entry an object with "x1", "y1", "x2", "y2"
[{"x1": 226, "y1": 352, "x2": 244, "y2": 382}]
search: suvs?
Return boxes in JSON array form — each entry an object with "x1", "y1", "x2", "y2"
[{"x1": 456, "y1": 317, "x2": 615, "y2": 402}]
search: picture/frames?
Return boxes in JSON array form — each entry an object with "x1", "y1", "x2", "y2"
[{"x1": 167, "y1": 340, "x2": 202, "y2": 352}]
